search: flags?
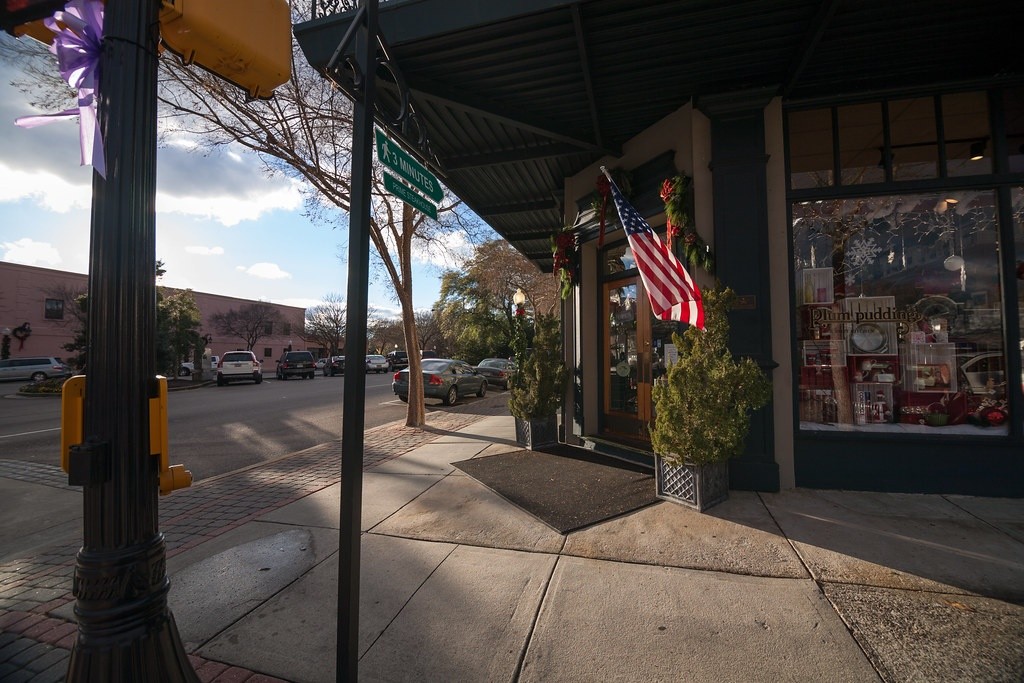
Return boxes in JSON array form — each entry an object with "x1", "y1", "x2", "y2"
[{"x1": 608, "y1": 179, "x2": 706, "y2": 332}]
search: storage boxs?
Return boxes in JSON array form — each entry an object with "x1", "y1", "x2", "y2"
[{"x1": 796, "y1": 267, "x2": 966, "y2": 424}]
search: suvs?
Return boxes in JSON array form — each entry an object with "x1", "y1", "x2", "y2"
[
  {"x1": 216, "y1": 349, "x2": 265, "y2": 387},
  {"x1": 275, "y1": 350, "x2": 316, "y2": 381}
]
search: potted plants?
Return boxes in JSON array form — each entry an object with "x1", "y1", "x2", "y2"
[
  {"x1": 646, "y1": 278, "x2": 772, "y2": 513},
  {"x1": 508, "y1": 302, "x2": 570, "y2": 450}
]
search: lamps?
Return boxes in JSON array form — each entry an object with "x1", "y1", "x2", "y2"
[
  {"x1": 878, "y1": 148, "x2": 896, "y2": 171},
  {"x1": 970, "y1": 140, "x2": 989, "y2": 160},
  {"x1": 944, "y1": 192, "x2": 960, "y2": 203}
]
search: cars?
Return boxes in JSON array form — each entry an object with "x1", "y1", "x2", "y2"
[
  {"x1": 179, "y1": 354, "x2": 220, "y2": 376},
  {"x1": 386, "y1": 350, "x2": 409, "y2": 371},
  {"x1": 419, "y1": 350, "x2": 437, "y2": 360},
  {"x1": 0, "y1": 355, "x2": 70, "y2": 385},
  {"x1": 315, "y1": 357, "x2": 330, "y2": 370},
  {"x1": 322, "y1": 354, "x2": 346, "y2": 378},
  {"x1": 392, "y1": 357, "x2": 489, "y2": 407},
  {"x1": 365, "y1": 353, "x2": 391, "y2": 374},
  {"x1": 943, "y1": 350, "x2": 1024, "y2": 398},
  {"x1": 472, "y1": 356, "x2": 517, "y2": 391}
]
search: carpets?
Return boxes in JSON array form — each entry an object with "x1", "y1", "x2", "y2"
[{"x1": 449, "y1": 443, "x2": 664, "y2": 535}]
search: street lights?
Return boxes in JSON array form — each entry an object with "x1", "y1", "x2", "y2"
[{"x1": 512, "y1": 287, "x2": 527, "y2": 390}]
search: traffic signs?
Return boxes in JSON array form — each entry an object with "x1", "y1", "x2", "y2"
[{"x1": 375, "y1": 126, "x2": 444, "y2": 205}]
